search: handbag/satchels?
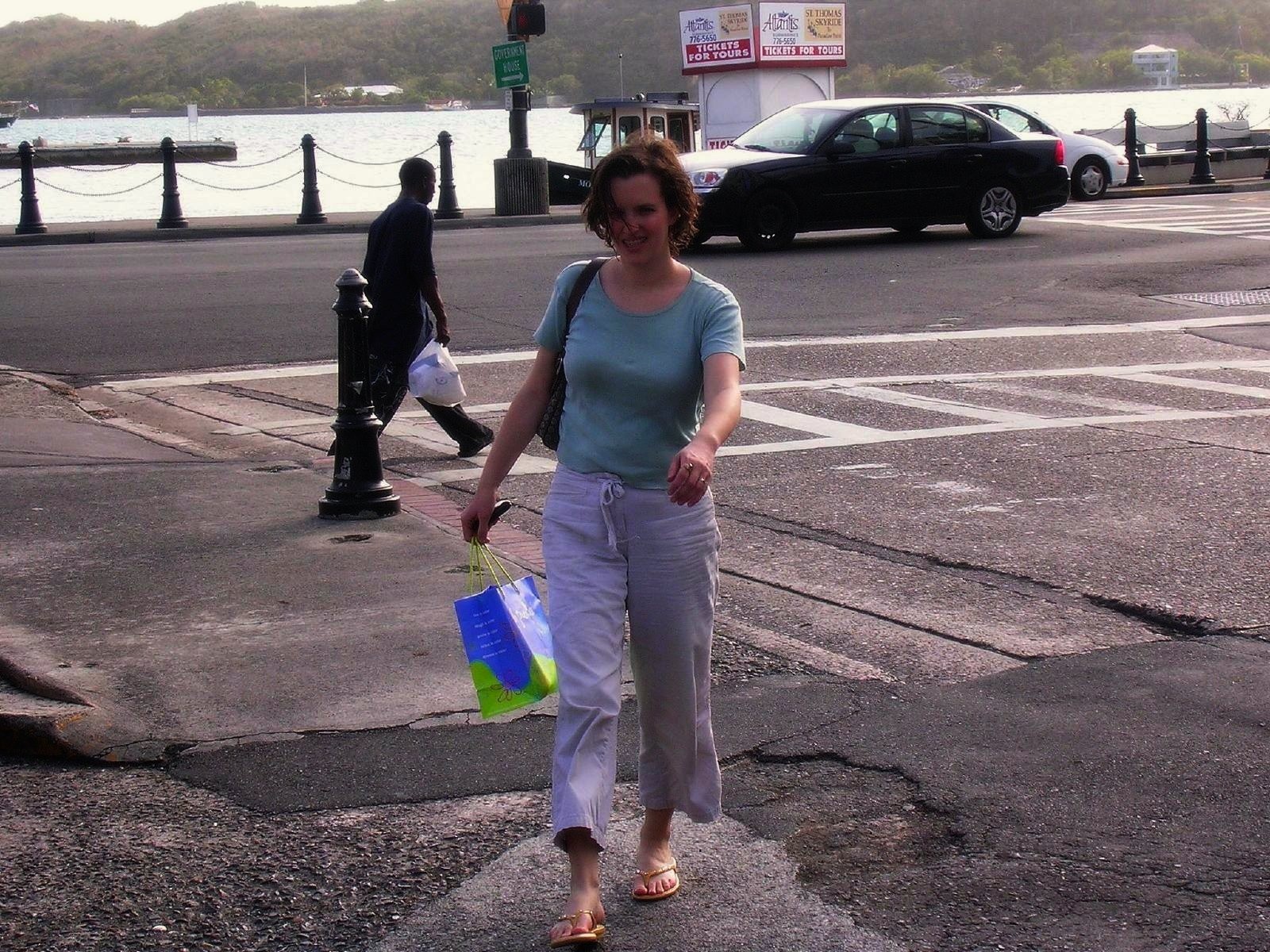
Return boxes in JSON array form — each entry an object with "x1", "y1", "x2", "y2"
[
  {"x1": 407, "y1": 338, "x2": 467, "y2": 407},
  {"x1": 537, "y1": 257, "x2": 613, "y2": 451},
  {"x1": 454, "y1": 536, "x2": 558, "y2": 719}
]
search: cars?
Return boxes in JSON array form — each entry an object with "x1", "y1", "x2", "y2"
[
  {"x1": 673, "y1": 96, "x2": 1069, "y2": 253},
  {"x1": 856, "y1": 99, "x2": 1130, "y2": 202}
]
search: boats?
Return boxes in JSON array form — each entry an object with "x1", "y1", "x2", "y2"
[
  {"x1": 425, "y1": 99, "x2": 471, "y2": 112},
  {"x1": 547, "y1": 91, "x2": 700, "y2": 206},
  {"x1": 0, "y1": 100, "x2": 29, "y2": 129}
]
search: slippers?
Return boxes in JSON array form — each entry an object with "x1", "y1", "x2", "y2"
[
  {"x1": 632, "y1": 857, "x2": 679, "y2": 900},
  {"x1": 550, "y1": 909, "x2": 606, "y2": 948}
]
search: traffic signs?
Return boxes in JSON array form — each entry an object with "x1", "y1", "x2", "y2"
[{"x1": 491, "y1": 39, "x2": 530, "y2": 89}]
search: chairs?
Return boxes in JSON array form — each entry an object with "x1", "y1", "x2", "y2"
[
  {"x1": 875, "y1": 128, "x2": 896, "y2": 141},
  {"x1": 852, "y1": 120, "x2": 873, "y2": 140}
]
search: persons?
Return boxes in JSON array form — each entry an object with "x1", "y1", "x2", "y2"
[
  {"x1": 460, "y1": 129, "x2": 746, "y2": 949},
  {"x1": 323, "y1": 158, "x2": 495, "y2": 457},
  {"x1": 839, "y1": 125, "x2": 881, "y2": 152}
]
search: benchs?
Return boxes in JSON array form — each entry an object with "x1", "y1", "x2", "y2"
[{"x1": 1074, "y1": 121, "x2": 1270, "y2": 185}]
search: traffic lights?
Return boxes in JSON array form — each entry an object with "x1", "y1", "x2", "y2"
[{"x1": 510, "y1": 3, "x2": 546, "y2": 36}]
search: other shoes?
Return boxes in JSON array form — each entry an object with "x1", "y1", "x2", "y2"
[{"x1": 457, "y1": 430, "x2": 495, "y2": 457}]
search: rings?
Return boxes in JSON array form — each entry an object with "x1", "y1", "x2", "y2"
[
  {"x1": 684, "y1": 462, "x2": 693, "y2": 472},
  {"x1": 699, "y1": 476, "x2": 705, "y2": 483}
]
